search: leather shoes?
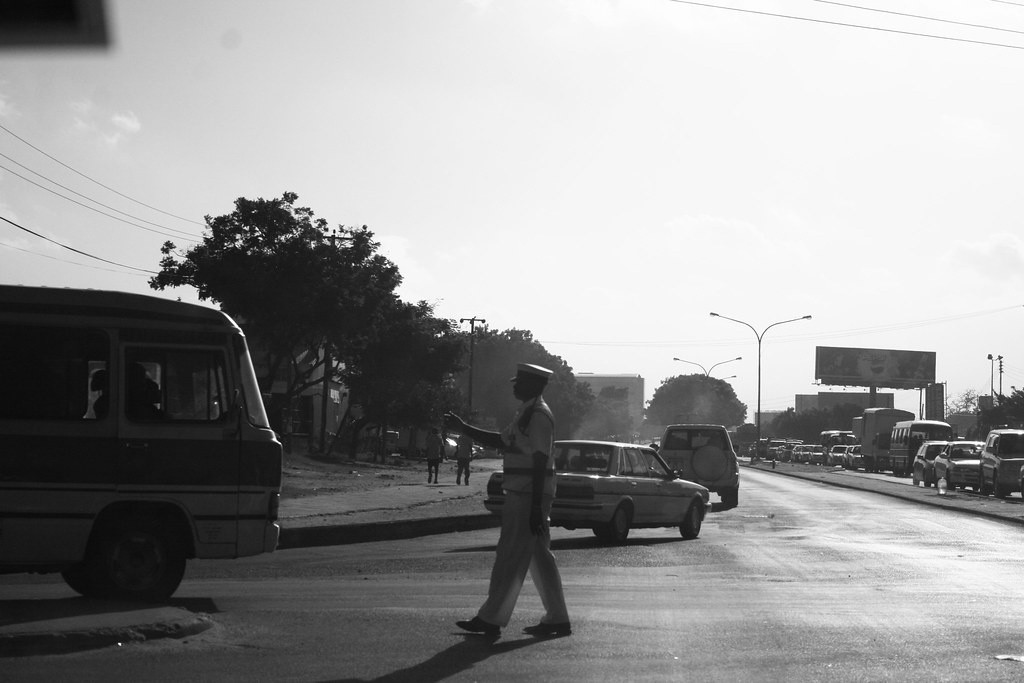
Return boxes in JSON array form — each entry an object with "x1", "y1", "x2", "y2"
[
  {"x1": 455, "y1": 614, "x2": 501, "y2": 635},
  {"x1": 524, "y1": 621, "x2": 572, "y2": 635}
]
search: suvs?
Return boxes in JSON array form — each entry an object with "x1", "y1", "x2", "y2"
[{"x1": 657, "y1": 424, "x2": 740, "y2": 507}]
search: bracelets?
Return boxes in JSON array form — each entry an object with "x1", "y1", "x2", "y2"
[{"x1": 532, "y1": 503, "x2": 542, "y2": 510}]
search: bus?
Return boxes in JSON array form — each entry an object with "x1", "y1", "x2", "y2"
[
  {"x1": 889, "y1": 420, "x2": 958, "y2": 476},
  {"x1": 0, "y1": 285, "x2": 283, "y2": 602}
]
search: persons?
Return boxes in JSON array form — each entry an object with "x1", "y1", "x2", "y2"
[
  {"x1": 443, "y1": 362, "x2": 572, "y2": 636},
  {"x1": 455, "y1": 433, "x2": 472, "y2": 486},
  {"x1": 425, "y1": 427, "x2": 444, "y2": 483},
  {"x1": 144, "y1": 378, "x2": 175, "y2": 421}
]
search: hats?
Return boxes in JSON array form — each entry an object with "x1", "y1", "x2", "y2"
[{"x1": 510, "y1": 362, "x2": 554, "y2": 385}]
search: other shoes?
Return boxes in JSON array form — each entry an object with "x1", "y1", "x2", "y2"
[
  {"x1": 457, "y1": 476, "x2": 460, "y2": 484},
  {"x1": 465, "y1": 481, "x2": 468, "y2": 485},
  {"x1": 428, "y1": 475, "x2": 432, "y2": 483},
  {"x1": 434, "y1": 481, "x2": 438, "y2": 484}
]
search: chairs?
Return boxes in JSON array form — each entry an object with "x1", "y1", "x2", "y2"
[
  {"x1": 570, "y1": 456, "x2": 590, "y2": 471},
  {"x1": 90, "y1": 368, "x2": 109, "y2": 420}
]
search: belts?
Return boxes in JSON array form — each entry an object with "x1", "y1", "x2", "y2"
[{"x1": 502, "y1": 465, "x2": 556, "y2": 478}]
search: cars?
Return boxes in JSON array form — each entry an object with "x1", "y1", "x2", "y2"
[
  {"x1": 981, "y1": 428, "x2": 1024, "y2": 499},
  {"x1": 849, "y1": 445, "x2": 866, "y2": 470},
  {"x1": 1018, "y1": 465, "x2": 1024, "y2": 499},
  {"x1": 384, "y1": 425, "x2": 484, "y2": 460},
  {"x1": 828, "y1": 444, "x2": 847, "y2": 467},
  {"x1": 843, "y1": 446, "x2": 854, "y2": 470},
  {"x1": 913, "y1": 441, "x2": 949, "y2": 487},
  {"x1": 934, "y1": 441, "x2": 985, "y2": 490},
  {"x1": 484, "y1": 440, "x2": 712, "y2": 546},
  {"x1": 728, "y1": 422, "x2": 856, "y2": 467}
]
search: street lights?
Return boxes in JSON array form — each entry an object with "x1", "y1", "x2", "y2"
[
  {"x1": 674, "y1": 356, "x2": 742, "y2": 378},
  {"x1": 719, "y1": 376, "x2": 736, "y2": 381},
  {"x1": 460, "y1": 316, "x2": 486, "y2": 424},
  {"x1": 988, "y1": 354, "x2": 1003, "y2": 407},
  {"x1": 710, "y1": 312, "x2": 811, "y2": 460}
]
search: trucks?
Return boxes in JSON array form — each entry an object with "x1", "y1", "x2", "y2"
[{"x1": 852, "y1": 416, "x2": 863, "y2": 444}]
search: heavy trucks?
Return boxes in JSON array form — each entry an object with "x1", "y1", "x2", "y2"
[{"x1": 859, "y1": 407, "x2": 915, "y2": 473}]
